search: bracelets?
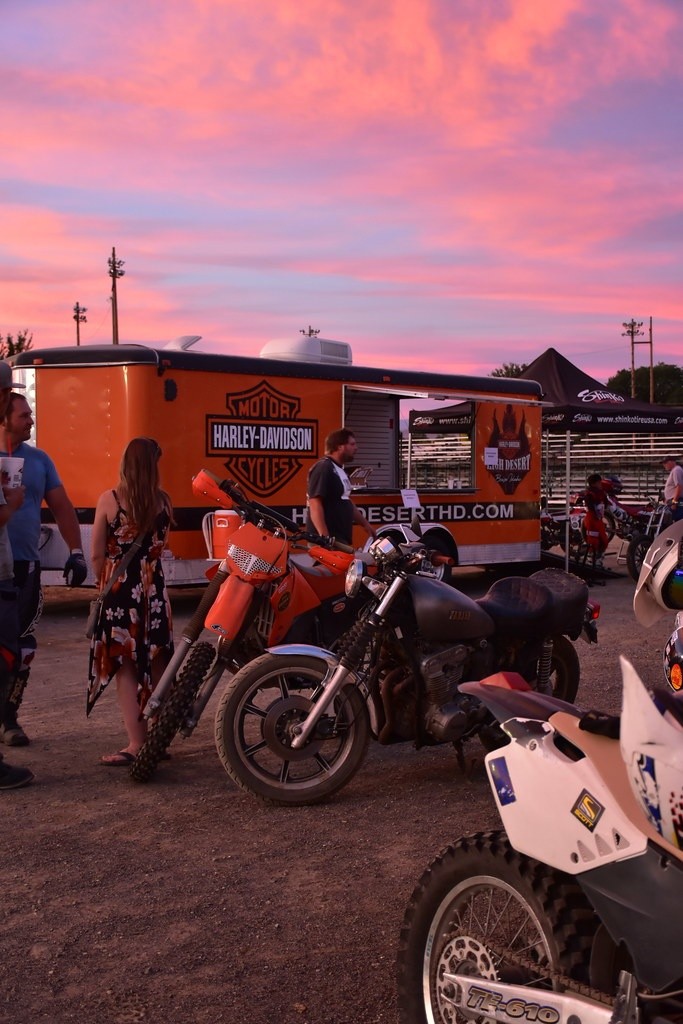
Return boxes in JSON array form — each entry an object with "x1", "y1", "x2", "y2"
[
  {"x1": 362, "y1": 521, "x2": 368, "y2": 527},
  {"x1": 672, "y1": 500, "x2": 678, "y2": 505}
]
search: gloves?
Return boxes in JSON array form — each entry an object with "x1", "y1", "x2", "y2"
[{"x1": 63, "y1": 553, "x2": 87, "y2": 587}]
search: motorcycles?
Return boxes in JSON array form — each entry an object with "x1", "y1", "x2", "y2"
[
  {"x1": 539, "y1": 485, "x2": 682, "y2": 585},
  {"x1": 394, "y1": 655, "x2": 682, "y2": 1023},
  {"x1": 128, "y1": 466, "x2": 380, "y2": 784},
  {"x1": 213, "y1": 533, "x2": 600, "y2": 809}
]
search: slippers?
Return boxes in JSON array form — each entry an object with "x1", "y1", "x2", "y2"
[{"x1": 100, "y1": 751, "x2": 135, "y2": 764}]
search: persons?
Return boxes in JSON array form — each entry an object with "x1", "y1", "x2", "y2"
[
  {"x1": 583, "y1": 475, "x2": 609, "y2": 563},
  {"x1": 306, "y1": 428, "x2": 378, "y2": 553},
  {"x1": 0, "y1": 360, "x2": 87, "y2": 790},
  {"x1": 658, "y1": 455, "x2": 683, "y2": 522},
  {"x1": 88, "y1": 436, "x2": 177, "y2": 766}
]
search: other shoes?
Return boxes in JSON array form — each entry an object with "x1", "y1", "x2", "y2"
[
  {"x1": 0, "y1": 756, "x2": 34, "y2": 789},
  {"x1": 0, "y1": 718, "x2": 30, "y2": 744}
]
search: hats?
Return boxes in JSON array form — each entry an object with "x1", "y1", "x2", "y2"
[
  {"x1": 0, "y1": 362, "x2": 26, "y2": 388},
  {"x1": 659, "y1": 456, "x2": 675, "y2": 463}
]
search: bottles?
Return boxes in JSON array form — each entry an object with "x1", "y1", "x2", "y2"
[{"x1": 668, "y1": 570, "x2": 683, "y2": 608}]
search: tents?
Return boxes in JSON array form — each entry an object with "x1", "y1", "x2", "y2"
[{"x1": 408, "y1": 346, "x2": 683, "y2": 575}]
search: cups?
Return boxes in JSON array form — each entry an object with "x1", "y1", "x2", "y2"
[{"x1": 0, "y1": 457, "x2": 25, "y2": 489}]
